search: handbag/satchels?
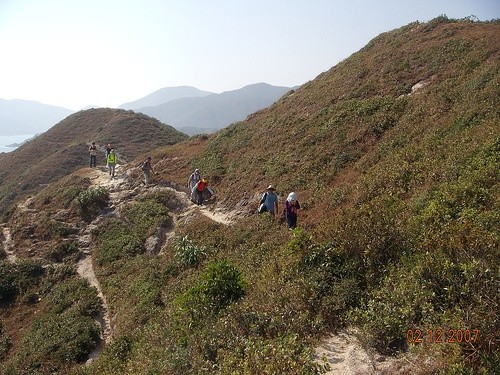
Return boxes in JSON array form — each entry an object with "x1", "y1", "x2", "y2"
[
  {"x1": 283, "y1": 208, "x2": 289, "y2": 218},
  {"x1": 142, "y1": 161, "x2": 147, "y2": 171},
  {"x1": 259, "y1": 203, "x2": 267, "y2": 212}
]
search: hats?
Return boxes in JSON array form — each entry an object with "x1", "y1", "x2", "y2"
[
  {"x1": 202, "y1": 178, "x2": 207, "y2": 183},
  {"x1": 286, "y1": 192, "x2": 296, "y2": 202},
  {"x1": 111, "y1": 149, "x2": 114, "y2": 152},
  {"x1": 146, "y1": 156, "x2": 152, "y2": 161},
  {"x1": 108, "y1": 144, "x2": 112, "y2": 147},
  {"x1": 195, "y1": 169, "x2": 199, "y2": 175},
  {"x1": 266, "y1": 185, "x2": 276, "y2": 191}
]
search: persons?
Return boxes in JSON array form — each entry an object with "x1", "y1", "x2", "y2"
[
  {"x1": 286, "y1": 191, "x2": 300, "y2": 231},
  {"x1": 142, "y1": 156, "x2": 153, "y2": 186},
  {"x1": 192, "y1": 176, "x2": 209, "y2": 206},
  {"x1": 89, "y1": 142, "x2": 98, "y2": 168},
  {"x1": 104, "y1": 143, "x2": 111, "y2": 169},
  {"x1": 107, "y1": 148, "x2": 117, "y2": 178},
  {"x1": 188, "y1": 168, "x2": 200, "y2": 204},
  {"x1": 259, "y1": 185, "x2": 278, "y2": 222}
]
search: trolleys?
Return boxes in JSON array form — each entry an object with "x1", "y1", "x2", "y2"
[{"x1": 189, "y1": 185, "x2": 217, "y2": 206}]
